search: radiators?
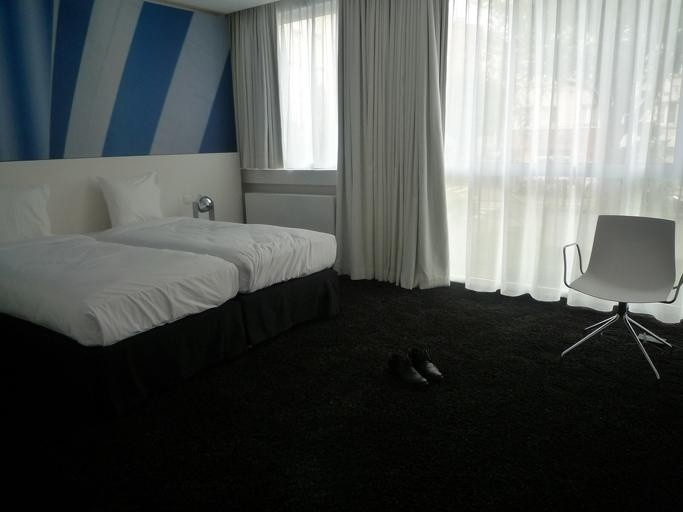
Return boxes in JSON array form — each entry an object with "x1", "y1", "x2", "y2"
[{"x1": 239, "y1": 190, "x2": 339, "y2": 239}]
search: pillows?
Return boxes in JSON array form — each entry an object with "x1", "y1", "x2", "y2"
[
  {"x1": 96, "y1": 169, "x2": 161, "y2": 228},
  {"x1": 0, "y1": 185, "x2": 54, "y2": 236}
]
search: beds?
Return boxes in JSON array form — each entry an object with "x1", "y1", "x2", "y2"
[
  {"x1": 85, "y1": 216, "x2": 341, "y2": 351},
  {"x1": 0, "y1": 235, "x2": 248, "y2": 402}
]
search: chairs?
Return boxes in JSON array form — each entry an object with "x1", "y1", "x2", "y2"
[{"x1": 557, "y1": 209, "x2": 681, "y2": 387}]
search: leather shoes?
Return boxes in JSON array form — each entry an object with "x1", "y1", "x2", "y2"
[
  {"x1": 385, "y1": 352, "x2": 428, "y2": 386},
  {"x1": 409, "y1": 344, "x2": 441, "y2": 377}
]
